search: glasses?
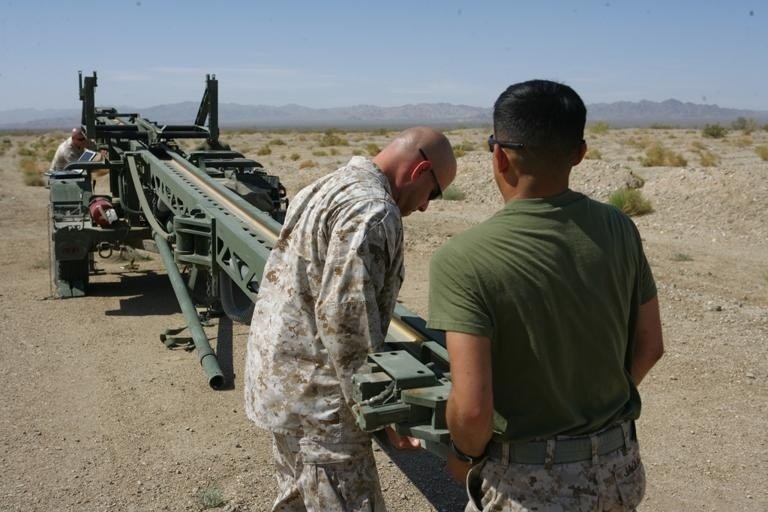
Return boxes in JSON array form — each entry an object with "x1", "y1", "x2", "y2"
[
  {"x1": 488, "y1": 135, "x2": 524, "y2": 150},
  {"x1": 418, "y1": 148, "x2": 443, "y2": 200}
]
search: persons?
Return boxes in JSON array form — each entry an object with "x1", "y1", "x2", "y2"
[
  {"x1": 49, "y1": 127, "x2": 106, "y2": 171},
  {"x1": 428, "y1": 80, "x2": 664, "y2": 512},
  {"x1": 244, "y1": 124, "x2": 457, "y2": 512}
]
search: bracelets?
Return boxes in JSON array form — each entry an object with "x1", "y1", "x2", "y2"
[{"x1": 449, "y1": 443, "x2": 483, "y2": 464}]
